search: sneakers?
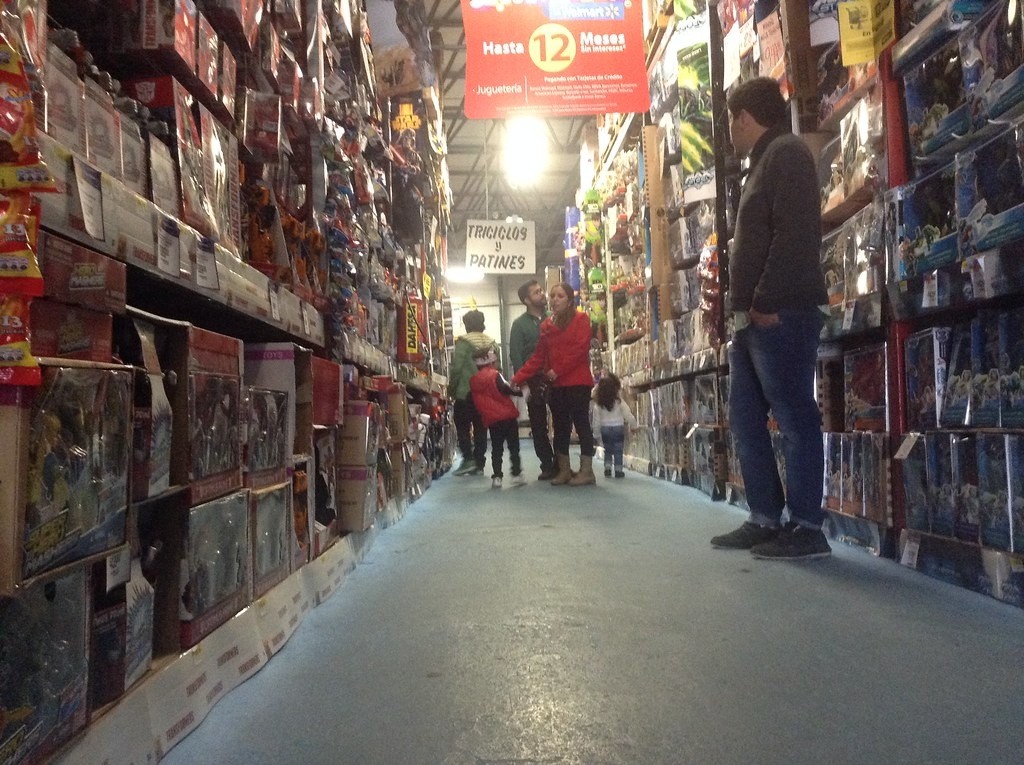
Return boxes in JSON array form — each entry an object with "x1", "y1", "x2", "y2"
[
  {"x1": 751, "y1": 524, "x2": 833, "y2": 561},
  {"x1": 492, "y1": 477, "x2": 503, "y2": 488},
  {"x1": 511, "y1": 473, "x2": 529, "y2": 485},
  {"x1": 711, "y1": 517, "x2": 785, "y2": 547},
  {"x1": 469, "y1": 468, "x2": 484, "y2": 478},
  {"x1": 452, "y1": 456, "x2": 476, "y2": 475}
]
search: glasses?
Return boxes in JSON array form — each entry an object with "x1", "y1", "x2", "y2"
[{"x1": 530, "y1": 287, "x2": 545, "y2": 296}]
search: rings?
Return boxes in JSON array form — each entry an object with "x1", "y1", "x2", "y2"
[{"x1": 550, "y1": 379, "x2": 552, "y2": 382}]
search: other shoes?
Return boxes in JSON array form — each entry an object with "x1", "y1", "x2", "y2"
[
  {"x1": 615, "y1": 472, "x2": 626, "y2": 478},
  {"x1": 604, "y1": 468, "x2": 613, "y2": 477},
  {"x1": 538, "y1": 469, "x2": 559, "y2": 480}
]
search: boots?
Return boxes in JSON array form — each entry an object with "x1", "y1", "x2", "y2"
[
  {"x1": 568, "y1": 456, "x2": 596, "y2": 486},
  {"x1": 551, "y1": 453, "x2": 571, "y2": 485}
]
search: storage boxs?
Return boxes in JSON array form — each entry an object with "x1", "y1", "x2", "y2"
[
  {"x1": 545, "y1": 265, "x2": 560, "y2": 292},
  {"x1": 665, "y1": 16, "x2": 1022, "y2": 554},
  {"x1": 1, "y1": 0, "x2": 415, "y2": 765},
  {"x1": 558, "y1": 266, "x2": 566, "y2": 283}
]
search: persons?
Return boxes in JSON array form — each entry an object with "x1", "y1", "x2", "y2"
[
  {"x1": 447, "y1": 310, "x2": 501, "y2": 476},
  {"x1": 510, "y1": 280, "x2": 559, "y2": 481},
  {"x1": 711, "y1": 78, "x2": 833, "y2": 560},
  {"x1": 510, "y1": 284, "x2": 595, "y2": 486},
  {"x1": 469, "y1": 348, "x2": 522, "y2": 487},
  {"x1": 592, "y1": 373, "x2": 638, "y2": 478}
]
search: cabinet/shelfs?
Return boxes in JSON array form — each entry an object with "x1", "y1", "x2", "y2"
[
  {"x1": 0, "y1": 0, "x2": 457, "y2": 765},
  {"x1": 575, "y1": 0, "x2": 1024, "y2": 608}
]
